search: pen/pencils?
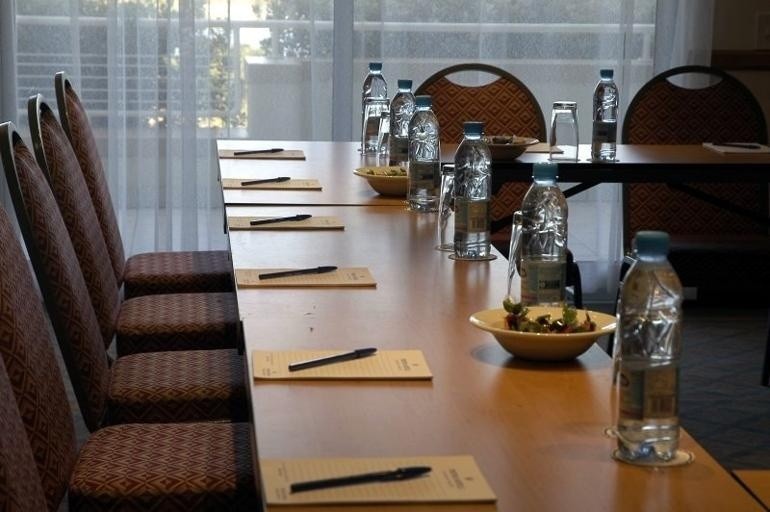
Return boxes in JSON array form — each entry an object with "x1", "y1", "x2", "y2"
[
  {"x1": 290, "y1": 464, "x2": 433, "y2": 490},
  {"x1": 712, "y1": 139, "x2": 762, "y2": 150},
  {"x1": 288, "y1": 348, "x2": 377, "y2": 368},
  {"x1": 233, "y1": 148, "x2": 283, "y2": 155},
  {"x1": 249, "y1": 214, "x2": 312, "y2": 225},
  {"x1": 240, "y1": 177, "x2": 291, "y2": 186},
  {"x1": 258, "y1": 265, "x2": 337, "y2": 280}
]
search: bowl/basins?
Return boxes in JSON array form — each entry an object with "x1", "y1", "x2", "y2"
[
  {"x1": 482, "y1": 134, "x2": 540, "y2": 162},
  {"x1": 467, "y1": 305, "x2": 620, "y2": 360},
  {"x1": 352, "y1": 164, "x2": 413, "y2": 195}
]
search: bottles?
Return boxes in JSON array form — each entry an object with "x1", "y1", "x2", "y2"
[
  {"x1": 361, "y1": 62, "x2": 389, "y2": 152},
  {"x1": 591, "y1": 68, "x2": 619, "y2": 162},
  {"x1": 519, "y1": 162, "x2": 570, "y2": 308},
  {"x1": 406, "y1": 94, "x2": 441, "y2": 213},
  {"x1": 451, "y1": 121, "x2": 493, "y2": 260},
  {"x1": 389, "y1": 79, "x2": 417, "y2": 167},
  {"x1": 613, "y1": 226, "x2": 684, "y2": 462}
]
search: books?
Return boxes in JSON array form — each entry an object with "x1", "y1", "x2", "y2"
[
  {"x1": 218, "y1": 150, "x2": 306, "y2": 160},
  {"x1": 236, "y1": 267, "x2": 376, "y2": 287},
  {"x1": 526, "y1": 141, "x2": 565, "y2": 153},
  {"x1": 260, "y1": 454, "x2": 498, "y2": 505},
  {"x1": 702, "y1": 142, "x2": 769, "y2": 155},
  {"x1": 222, "y1": 178, "x2": 323, "y2": 190},
  {"x1": 251, "y1": 348, "x2": 434, "y2": 381},
  {"x1": 228, "y1": 215, "x2": 345, "y2": 230}
]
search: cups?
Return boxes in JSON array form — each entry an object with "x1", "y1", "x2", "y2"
[
  {"x1": 547, "y1": 101, "x2": 579, "y2": 161},
  {"x1": 375, "y1": 111, "x2": 391, "y2": 168},
  {"x1": 505, "y1": 210, "x2": 527, "y2": 308},
  {"x1": 609, "y1": 297, "x2": 622, "y2": 434},
  {"x1": 360, "y1": 97, "x2": 391, "y2": 155},
  {"x1": 437, "y1": 163, "x2": 457, "y2": 251}
]
